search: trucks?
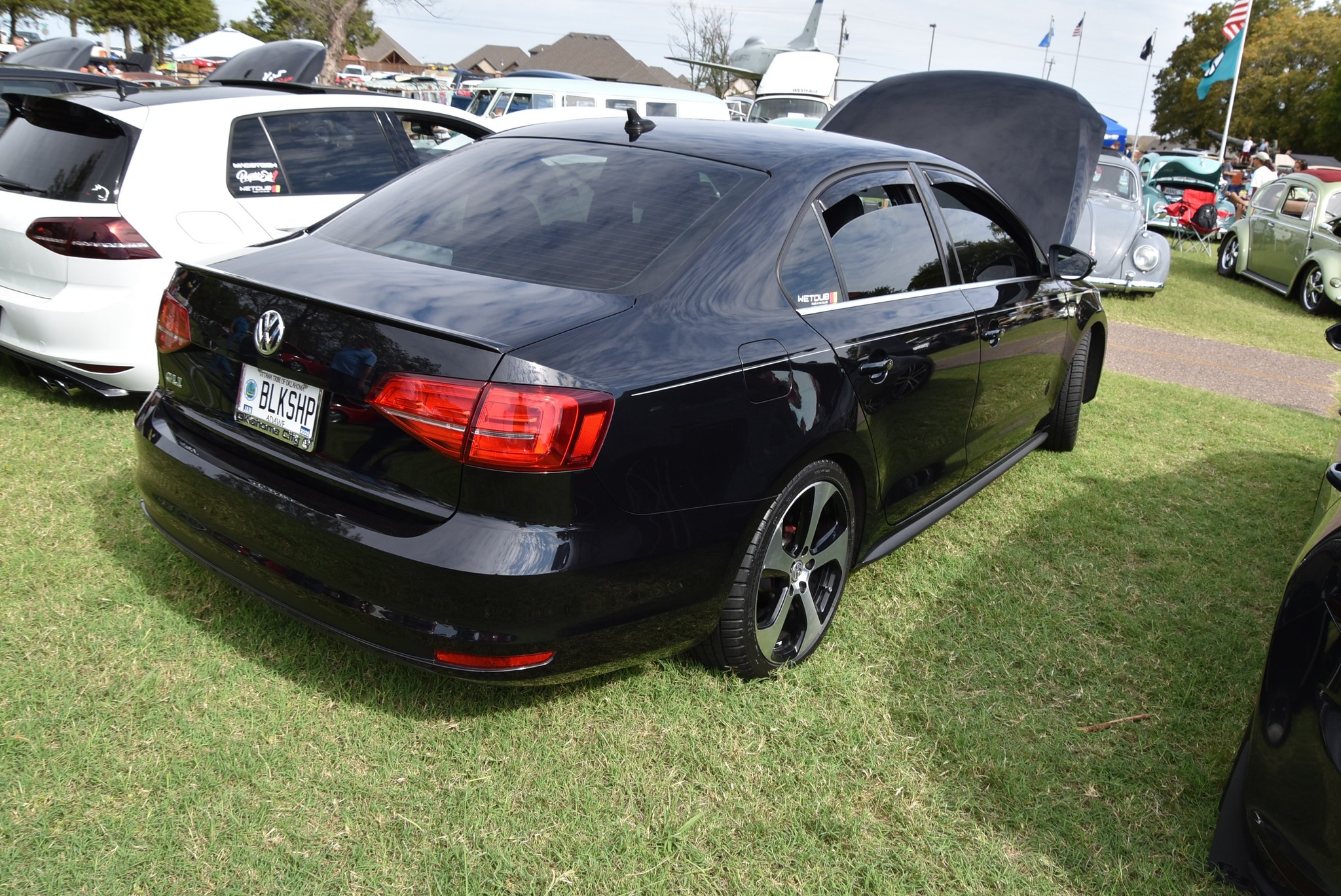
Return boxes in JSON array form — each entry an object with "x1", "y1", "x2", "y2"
[{"x1": 722, "y1": 95, "x2": 838, "y2": 131}]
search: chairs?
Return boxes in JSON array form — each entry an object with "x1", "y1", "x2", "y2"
[{"x1": 1163, "y1": 189, "x2": 1235, "y2": 257}]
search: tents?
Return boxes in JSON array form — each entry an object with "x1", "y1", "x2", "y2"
[{"x1": 170, "y1": 27, "x2": 266, "y2": 81}]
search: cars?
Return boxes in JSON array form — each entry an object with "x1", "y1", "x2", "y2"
[
  {"x1": 134, "y1": 68, "x2": 1108, "y2": 698},
  {"x1": 1070, "y1": 147, "x2": 1172, "y2": 299},
  {"x1": 1216, "y1": 169, "x2": 1341, "y2": 317},
  {"x1": 0, "y1": 38, "x2": 496, "y2": 410},
  {"x1": 1118, "y1": 150, "x2": 1236, "y2": 243},
  {"x1": 1207, "y1": 320, "x2": 1341, "y2": 896},
  {"x1": 31, "y1": 43, "x2": 599, "y2": 150}
]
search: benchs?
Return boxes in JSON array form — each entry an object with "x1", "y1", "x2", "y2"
[{"x1": 451, "y1": 192, "x2": 712, "y2": 287}]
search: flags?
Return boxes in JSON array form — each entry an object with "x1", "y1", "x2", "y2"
[
  {"x1": 1140, "y1": 33, "x2": 1155, "y2": 61},
  {"x1": 1038, "y1": 27, "x2": 1054, "y2": 47},
  {"x1": 1197, "y1": 27, "x2": 1244, "y2": 100},
  {"x1": 1072, "y1": 18, "x2": 1083, "y2": 37},
  {"x1": 1221, "y1": 0, "x2": 1250, "y2": 41}
]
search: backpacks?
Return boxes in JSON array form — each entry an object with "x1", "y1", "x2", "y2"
[{"x1": 1190, "y1": 203, "x2": 1217, "y2": 235}]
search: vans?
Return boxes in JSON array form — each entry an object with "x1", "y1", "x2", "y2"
[{"x1": 460, "y1": 76, "x2": 731, "y2": 152}]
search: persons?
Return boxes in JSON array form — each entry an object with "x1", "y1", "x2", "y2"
[
  {"x1": 1250, "y1": 151, "x2": 1278, "y2": 200},
  {"x1": 10, "y1": 34, "x2": 123, "y2": 75},
  {"x1": 1258, "y1": 142, "x2": 1269, "y2": 153},
  {"x1": 1219, "y1": 156, "x2": 1239, "y2": 204},
  {"x1": 1225, "y1": 172, "x2": 1251, "y2": 222},
  {"x1": 1239, "y1": 136, "x2": 1253, "y2": 164}
]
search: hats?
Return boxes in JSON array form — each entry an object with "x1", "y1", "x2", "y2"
[
  {"x1": 1242, "y1": 173, "x2": 1251, "y2": 179},
  {"x1": 1251, "y1": 152, "x2": 1271, "y2": 162}
]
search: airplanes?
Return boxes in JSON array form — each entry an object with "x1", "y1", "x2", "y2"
[{"x1": 663, "y1": 0, "x2": 876, "y2": 95}]
